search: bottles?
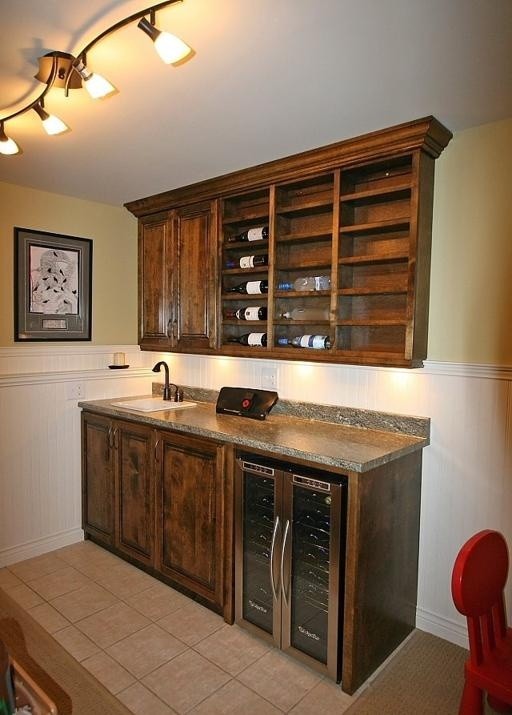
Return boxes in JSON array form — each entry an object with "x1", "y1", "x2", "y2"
[
  {"x1": 278, "y1": 275, "x2": 331, "y2": 292},
  {"x1": 224, "y1": 253, "x2": 268, "y2": 269},
  {"x1": 278, "y1": 305, "x2": 331, "y2": 321},
  {"x1": 227, "y1": 226, "x2": 269, "y2": 243},
  {"x1": 225, "y1": 306, "x2": 267, "y2": 321},
  {"x1": 226, "y1": 331, "x2": 267, "y2": 346},
  {"x1": 223, "y1": 279, "x2": 268, "y2": 295},
  {"x1": 276, "y1": 333, "x2": 331, "y2": 350}
]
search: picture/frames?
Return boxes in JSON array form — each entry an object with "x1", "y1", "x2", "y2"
[{"x1": 14, "y1": 226, "x2": 93, "y2": 342}]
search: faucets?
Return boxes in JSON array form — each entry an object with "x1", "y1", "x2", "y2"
[{"x1": 152, "y1": 361, "x2": 171, "y2": 400}]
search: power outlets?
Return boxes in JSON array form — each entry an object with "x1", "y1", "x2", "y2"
[{"x1": 65, "y1": 384, "x2": 85, "y2": 401}]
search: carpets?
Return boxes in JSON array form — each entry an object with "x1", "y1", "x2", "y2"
[
  {"x1": 0, "y1": 586, "x2": 136, "y2": 715},
  {"x1": 342, "y1": 627, "x2": 512, "y2": 715}
]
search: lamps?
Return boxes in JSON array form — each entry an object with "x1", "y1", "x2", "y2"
[{"x1": 0, "y1": 0, "x2": 192, "y2": 156}]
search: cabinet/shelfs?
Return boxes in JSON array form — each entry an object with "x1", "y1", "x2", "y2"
[
  {"x1": 217, "y1": 148, "x2": 435, "y2": 369},
  {"x1": 155, "y1": 429, "x2": 225, "y2": 618},
  {"x1": 138, "y1": 197, "x2": 218, "y2": 355},
  {"x1": 234, "y1": 453, "x2": 349, "y2": 686},
  {"x1": 81, "y1": 412, "x2": 153, "y2": 578}
]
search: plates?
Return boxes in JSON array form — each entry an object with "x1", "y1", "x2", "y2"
[{"x1": 107, "y1": 365, "x2": 129, "y2": 369}]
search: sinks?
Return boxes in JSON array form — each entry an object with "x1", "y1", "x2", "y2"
[{"x1": 110, "y1": 398, "x2": 197, "y2": 412}]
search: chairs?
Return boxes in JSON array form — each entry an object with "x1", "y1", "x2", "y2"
[{"x1": 451, "y1": 529, "x2": 512, "y2": 715}]
